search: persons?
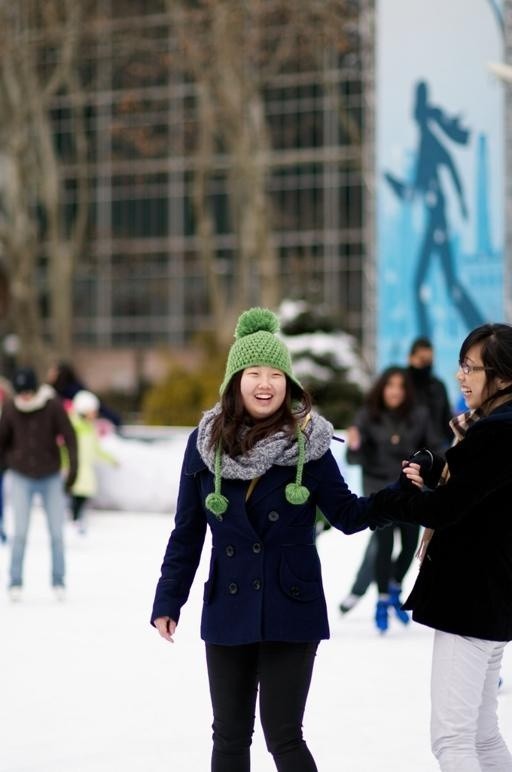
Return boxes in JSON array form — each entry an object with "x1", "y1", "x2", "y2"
[
  {"x1": 403, "y1": 323, "x2": 512, "y2": 772},
  {"x1": 337, "y1": 335, "x2": 454, "y2": 630},
  {"x1": 0, "y1": 340, "x2": 131, "y2": 606},
  {"x1": 150, "y1": 305, "x2": 424, "y2": 771}
]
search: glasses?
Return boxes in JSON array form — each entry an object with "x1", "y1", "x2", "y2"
[{"x1": 458, "y1": 359, "x2": 496, "y2": 377}]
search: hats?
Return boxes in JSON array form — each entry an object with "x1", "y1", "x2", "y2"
[{"x1": 205, "y1": 307, "x2": 311, "y2": 517}]
